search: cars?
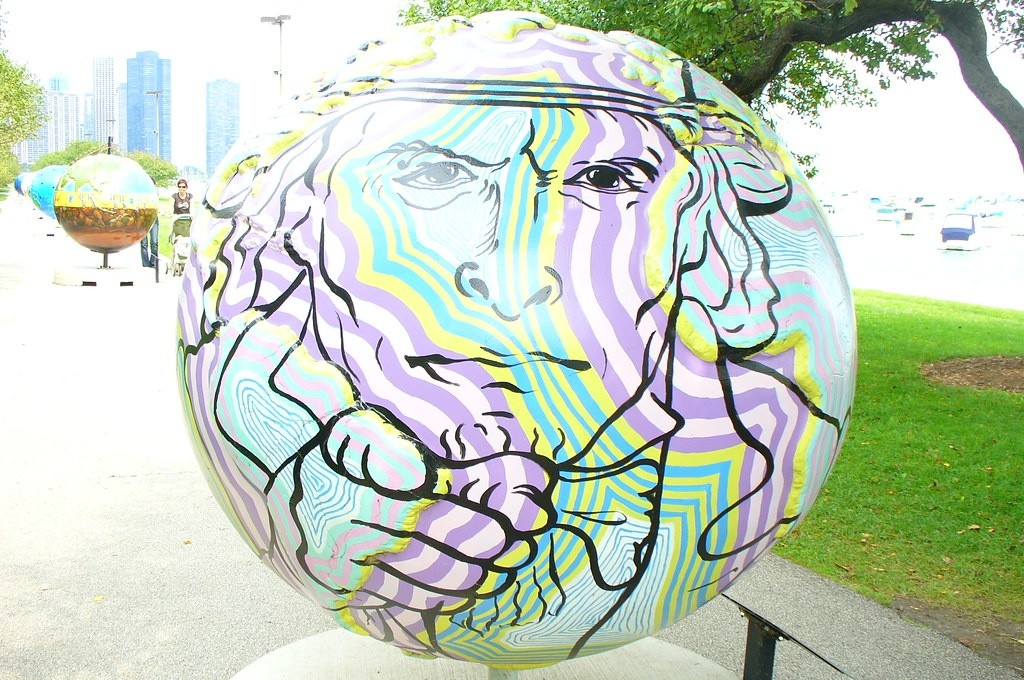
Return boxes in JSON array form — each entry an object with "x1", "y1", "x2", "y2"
[{"x1": 822, "y1": 194, "x2": 1024, "y2": 252}]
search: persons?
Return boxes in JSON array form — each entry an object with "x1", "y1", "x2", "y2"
[
  {"x1": 175, "y1": 234, "x2": 190, "y2": 260},
  {"x1": 169, "y1": 178, "x2": 195, "y2": 217}
]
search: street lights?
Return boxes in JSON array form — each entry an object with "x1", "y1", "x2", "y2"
[
  {"x1": 106, "y1": 118, "x2": 116, "y2": 142},
  {"x1": 84, "y1": 132, "x2": 93, "y2": 138},
  {"x1": 259, "y1": 14, "x2": 292, "y2": 98},
  {"x1": 145, "y1": 91, "x2": 164, "y2": 157}
]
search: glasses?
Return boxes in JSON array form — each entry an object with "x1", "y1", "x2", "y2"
[{"x1": 177, "y1": 186, "x2": 186, "y2": 188}]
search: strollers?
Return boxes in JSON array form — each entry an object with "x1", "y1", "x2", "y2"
[{"x1": 165, "y1": 212, "x2": 195, "y2": 277}]
